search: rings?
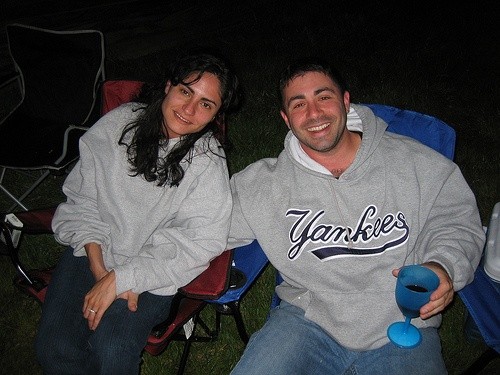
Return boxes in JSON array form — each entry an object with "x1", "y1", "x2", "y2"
[{"x1": 87, "y1": 308, "x2": 98, "y2": 314}]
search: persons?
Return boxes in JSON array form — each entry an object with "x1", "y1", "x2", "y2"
[
  {"x1": 83, "y1": 60, "x2": 486, "y2": 375},
  {"x1": 34, "y1": 50, "x2": 238, "y2": 375}
]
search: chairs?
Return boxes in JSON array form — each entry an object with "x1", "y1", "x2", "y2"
[
  {"x1": 0, "y1": 79, "x2": 234, "y2": 375},
  {"x1": 458, "y1": 203, "x2": 500, "y2": 375},
  {"x1": 204, "y1": 104, "x2": 457, "y2": 346},
  {"x1": 0, "y1": 24, "x2": 105, "y2": 213}
]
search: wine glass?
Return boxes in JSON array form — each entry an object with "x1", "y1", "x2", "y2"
[{"x1": 387, "y1": 265, "x2": 440, "y2": 349}]
